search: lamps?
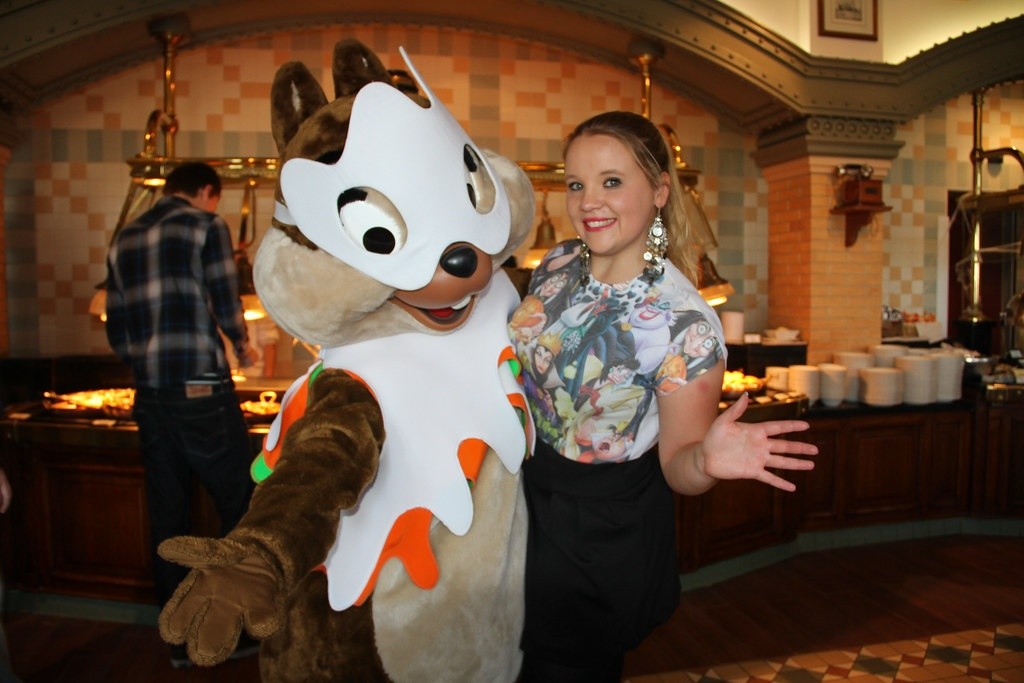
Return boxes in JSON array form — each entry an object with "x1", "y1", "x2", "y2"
[
  {"x1": 234, "y1": 182, "x2": 265, "y2": 321},
  {"x1": 524, "y1": 189, "x2": 558, "y2": 270},
  {"x1": 89, "y1": 182, "x2": 136, "y2": 322},
  {"x1": 694, "y1": 253, "x2": 736, "y2": 309}
]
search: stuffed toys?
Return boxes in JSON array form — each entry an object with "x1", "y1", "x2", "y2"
[{"x1": 156, "y1": 39, "x2": 536, "y2": 683}]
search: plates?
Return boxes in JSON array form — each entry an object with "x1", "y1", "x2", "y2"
[
  {"x1": 239, "y1": 401, "x2": 280, "y2": 420},
  {"x1": 719, "y1": 309, "x2": 806, "y2": 344},
  {"x1": 765, "y1": 343, "x2": 964, "y2": 408},
  {"x1": 721, "y1": 384, "x2": 765, "y2": 399}
]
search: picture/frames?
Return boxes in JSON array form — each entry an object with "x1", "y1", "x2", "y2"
[{"x1": 818, "y1": 0, "x2": 880, "y2": 43}]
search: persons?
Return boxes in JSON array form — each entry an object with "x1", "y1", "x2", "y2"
[
  {"x1": 508, "y1": 110, "x2": 818, "y2": 683},
  {"x1": 107, "y1": 163, "x2": 263, "y2": 666}
]
